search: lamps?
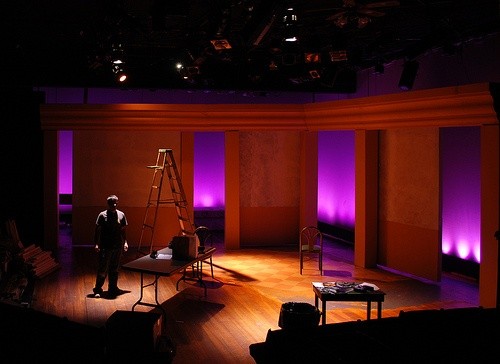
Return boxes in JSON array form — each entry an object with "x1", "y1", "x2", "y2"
[{"x1": 111, "y1": 0, "x2": 419, "y2": 90}]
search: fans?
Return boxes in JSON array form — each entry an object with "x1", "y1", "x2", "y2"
[{"x1": 306, "y1": 0, "x2": 402, "y2": 25}]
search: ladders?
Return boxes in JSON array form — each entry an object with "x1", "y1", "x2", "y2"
[{"x1": 137, "y1": 149, "x2": 196, "y2": 265}]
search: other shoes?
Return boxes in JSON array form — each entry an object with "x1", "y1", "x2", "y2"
[
  {"x1": 94, "y1": 292, "x2": 101, "y2": 298},
  {"x1": 108, "y1": 288, "x2": 123, "y2": 294}
]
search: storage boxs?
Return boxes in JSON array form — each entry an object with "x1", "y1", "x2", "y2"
[{"x1": 173, "y1": 236, "x2": 198, "y2": 261}]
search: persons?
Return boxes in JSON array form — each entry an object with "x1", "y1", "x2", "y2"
[{"x1": 93, "y1": 194, "x2": 129, "y2": 298}]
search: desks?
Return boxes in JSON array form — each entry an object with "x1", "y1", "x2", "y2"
[
  {"x1": 312, "y1": 282, "x2": 387, "y2": 324},
  {"x1": 121, "y1": 246, "x2": 216, "y2": 333}
]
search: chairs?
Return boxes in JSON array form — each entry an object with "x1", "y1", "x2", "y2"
[{"x1": 299, "y1": 226, "x2": 323, "y2": 275}]
